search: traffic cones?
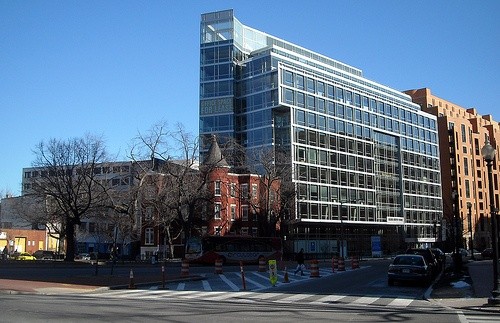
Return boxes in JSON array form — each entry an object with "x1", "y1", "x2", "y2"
[
  {"x1": 127, "y1": 268, "x2": 136, "y2": 289},
  {"x1": 281, "y1": 265, "x2": 290, "y2": 283}
]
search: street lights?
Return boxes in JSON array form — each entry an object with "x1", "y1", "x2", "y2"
[{"x1": 481, "y1": 134, "x2": 500, "y2": 306}]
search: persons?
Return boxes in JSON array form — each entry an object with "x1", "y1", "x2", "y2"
[
  {"x1": 3, "y1": 246, "x2": 8, "y2": 257},
  {"x1": 294, "y1": 248, "x2": 305, "y2": 275}
]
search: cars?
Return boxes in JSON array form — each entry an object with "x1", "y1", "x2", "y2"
[
  {"x1": 74, "y1": 252, "x2": 107, "y2": 265},
  {"x1": 402, "y1": 248, "x2": 446, "y2": 275},
  {"x1": 388, "y1": 254, "x2": 428, "y2": 286},
  {"x1": 472, "y1": 248, "x2": 493, "y2": 261},
  {"x1": 11, "y1": 250, "x2": 54, "y2": 261}
]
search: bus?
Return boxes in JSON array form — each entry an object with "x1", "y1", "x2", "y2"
[{"x1": 185, "y1": 234, "x2": 283, "y2": 266}]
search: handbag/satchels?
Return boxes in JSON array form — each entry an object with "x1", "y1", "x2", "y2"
[
  {"x1": 301, "y1": 264, "x2": 307, "y2": 271},
  {"x1": 297, "y1": 264, "x2": 300, "y2": 270}
]
